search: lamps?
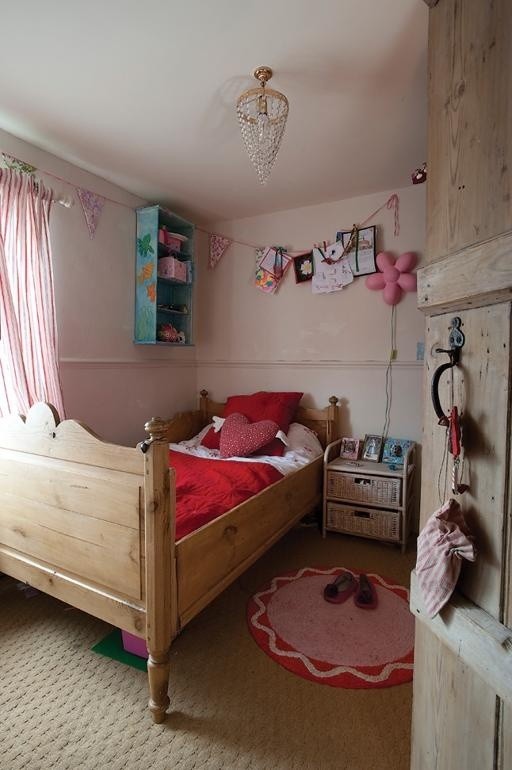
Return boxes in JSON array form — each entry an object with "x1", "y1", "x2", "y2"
[{"x1": 236, "y1": 66, "x2": 290, "y2": 186}]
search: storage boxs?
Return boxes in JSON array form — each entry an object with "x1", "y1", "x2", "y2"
[{"x1": 121, "y1": 630, "x2": 148, "y2": 659}]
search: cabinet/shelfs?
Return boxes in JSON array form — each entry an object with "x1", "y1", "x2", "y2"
[{"x1": 132, "y1": 204, "x2": 196, "y2": 346}]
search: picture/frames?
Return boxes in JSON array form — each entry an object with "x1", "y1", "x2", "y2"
[{"x1": 340, "y1": 434, "x2": 412, "y2": 465}]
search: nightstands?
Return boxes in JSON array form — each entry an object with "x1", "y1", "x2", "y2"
[{"x1": 322, "y1": 437, "x2": 418, "y2": 555}]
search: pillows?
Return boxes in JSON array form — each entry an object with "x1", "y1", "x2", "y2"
[{"x1": 200, "y1": 392, "x2": 304, "y2": 457}]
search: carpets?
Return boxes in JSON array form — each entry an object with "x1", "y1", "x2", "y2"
[
  {"x1": 245, "y1": 561, "x2": 415, "y2": 689},
  {"x1": 90, "y1": 629, "x2": 147, "y2": 672}
]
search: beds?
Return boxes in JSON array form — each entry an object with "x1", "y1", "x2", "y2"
[{"x1": 0, "y1": 388, "x2": 340, "y2": 724}]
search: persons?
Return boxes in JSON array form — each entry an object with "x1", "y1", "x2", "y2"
[{"x1": 368, "y1": 439, "x2": 375, "y2": 456}]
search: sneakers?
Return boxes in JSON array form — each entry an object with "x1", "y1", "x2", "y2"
[
  {"x1": 354, "y1": 573, "x2": 378, "y2": 608},
  {"x1": 325, "y1": 572, "x2": 357, "y2": 604}
]
